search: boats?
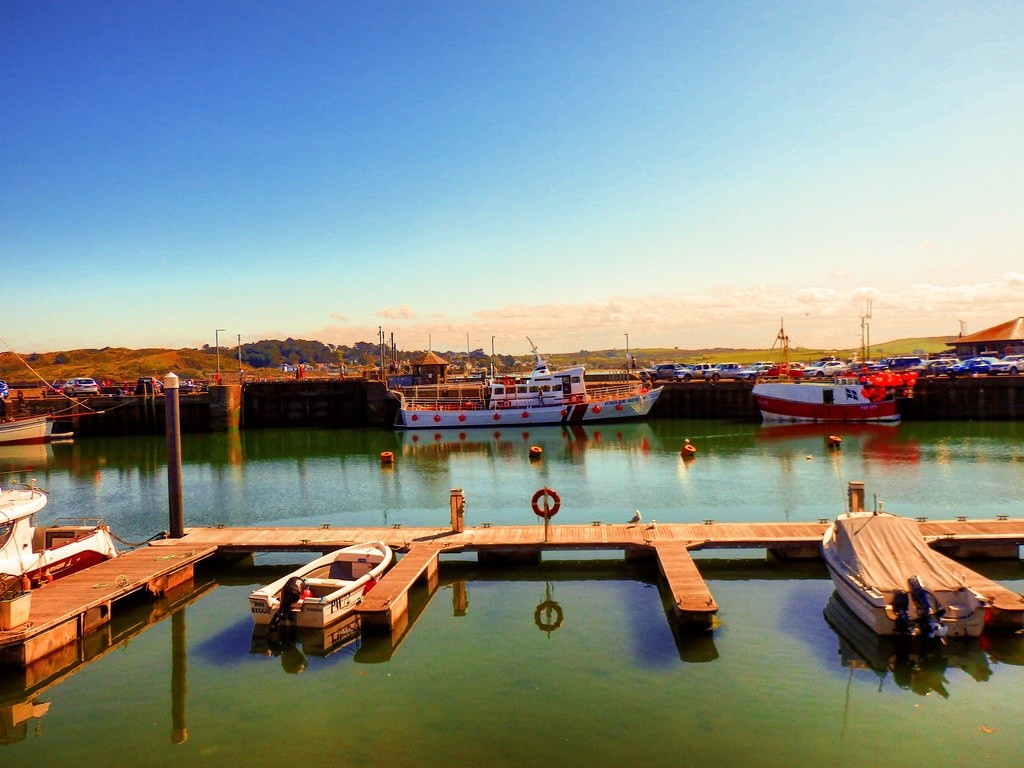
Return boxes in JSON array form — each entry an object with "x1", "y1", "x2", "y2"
[
  {"x1": 249, "y1": 606, "x2": 363, "y2": 658},
  {"x1": 750, "y1": 297, "x2": 903, "y2": 423},
  {"x1": 386, "y1": 335, "x2": 666, "y2": 431},
  {"x1": 0, "y1": 477, "x2": 126, "y2": 606},
  {"x1": 248, "y1": 538, "x2": 394, "y2": 633},
  {"x1": 0, "y1": 398, "x2": 106, "y2": 447},
  {"x1": 822, "y1": 587, "x2": 1024, "y2": 693},
  {"x1": 819, "y1": 492, "x2": 1024, "y2": 643}
]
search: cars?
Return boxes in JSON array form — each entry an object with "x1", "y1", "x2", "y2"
[
  {"x1": 802, "y1": 360, "x2": 846, "y2": 379},
  {"x1": 133, "y1": 376, "x2": 211, "y2": 395},
  {"x1": 0, "y1": 380, "x2": 10, "y2": 400},
  {"x1": 63, "y1": 377, "x2": 101, "y2": 397},
  {"x1": 840, "y1": 356, "x2": 1001, "y2": 378},
  {"x1": 990, "y1": 354, "x2": 1024, "y2": 377},
  {"x1": 41, "y1": 377, "x2": 68, "y2": 398},
  {"x1": 640, "y1": 361, "x2": 814, "y2": 382}
]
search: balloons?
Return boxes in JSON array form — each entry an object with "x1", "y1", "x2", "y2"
[
  {"x1": 861, "y1": 387, "x2": 886, "y2": 402},
  {"x1": 861, "y1": 373, "x2": 919, "y2": 387}
]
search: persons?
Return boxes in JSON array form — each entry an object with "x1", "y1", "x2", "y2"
[
  {"x1": 239, "y1": 368, "x2": 244, "y2": 383},
  {"x1": 632, "y1": 356, "x2": 636, "y2": 374},
  {"x1": 296, "y1": 366, "x2": 304, "y2": 382},
  {"x1": 101, "y1": 381, "x2": 105, "y2": 386},
  {"x1": 538, "y1": 387, "x2": 545, "y2": 406},
  {"x1": 182, "y1": 379, "x2": 202, "y2": 394},
  {"x1": 117, "y1": 383, "x2": 132, "y2": 396}
]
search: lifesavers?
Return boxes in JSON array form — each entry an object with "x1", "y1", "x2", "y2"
[
  {"x1": 569, "y1": 395, "x2": 578, "y2": 404},
  {"x1": 532, "y1": 489, "x2": 560, "y2": 517},
  {"x1": 504, "y1": 380, "x2": 509, "y2": 385},
  {"x1": 535, "y1": 601, "x2": 563, "y2": 631},
  {"x1": 503, "y1": 400, "x2": 511, "y2": 409}
]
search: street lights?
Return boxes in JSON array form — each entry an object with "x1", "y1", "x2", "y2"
[
  {"x1": 216, "y1": 329, "x2": 226, "y2": 379},
  {"x1": 624, "y1": 333, "x2": 629, "y2": 373}
]
